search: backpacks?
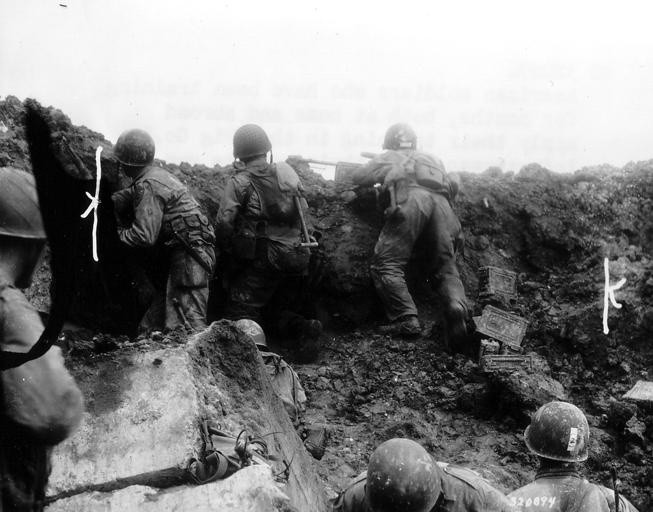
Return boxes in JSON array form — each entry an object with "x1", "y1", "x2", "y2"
[{"x1": 238, "y1": 166, "x2": 309, "y2": 224}]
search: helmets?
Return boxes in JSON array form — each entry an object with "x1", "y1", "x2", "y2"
[
  {"x1": 365, "y1": 437, "x2": 442, "y2": 512},
  {"x1": 0, "y1": 167, "x2": 50, "y2": 240},
  {"x1": 381, "y1": 123, "x2": 417, "y2": 150},
  {"x1": 236, "y1": 318, "x2": 269, "y2": 348},
  {"x1": 232, "y1": 123, "x2": 272, "y2": 158},
  {"x1": 112, "y1": 127, "x2": 154, "y2": 168},
  {"x1": 523, "y1": 399, "x2": 591, "y2": 462}
]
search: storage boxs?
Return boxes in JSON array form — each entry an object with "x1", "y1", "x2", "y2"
[
  {"x1": 474, "y1": 304, "x2": 531, "y2": 352},
  {"x1": 477, "y1": 265, "x2": 516, "y2": 299},
  {"x1": 478, "y1": 353, "x2": 534, "y2": 375}
]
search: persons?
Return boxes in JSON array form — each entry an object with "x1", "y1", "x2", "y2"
[{"x1": 0, "y1": 122, "x2": 639, "y2": 512}]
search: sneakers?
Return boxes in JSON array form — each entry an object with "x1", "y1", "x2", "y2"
[
  {"x1": 377, "y1": 318, "x2": 421, "y2": 336},
  {"x1": 445, "y1": 304, "x2": 468, "y2": 341},
  {"x1": 297, "y1": 319, "x2": 322, "y2": 364}
]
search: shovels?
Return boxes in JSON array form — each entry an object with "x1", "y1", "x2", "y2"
[{"x1": 270, "y1": 161, "x2": 319, "y2": 248}]
